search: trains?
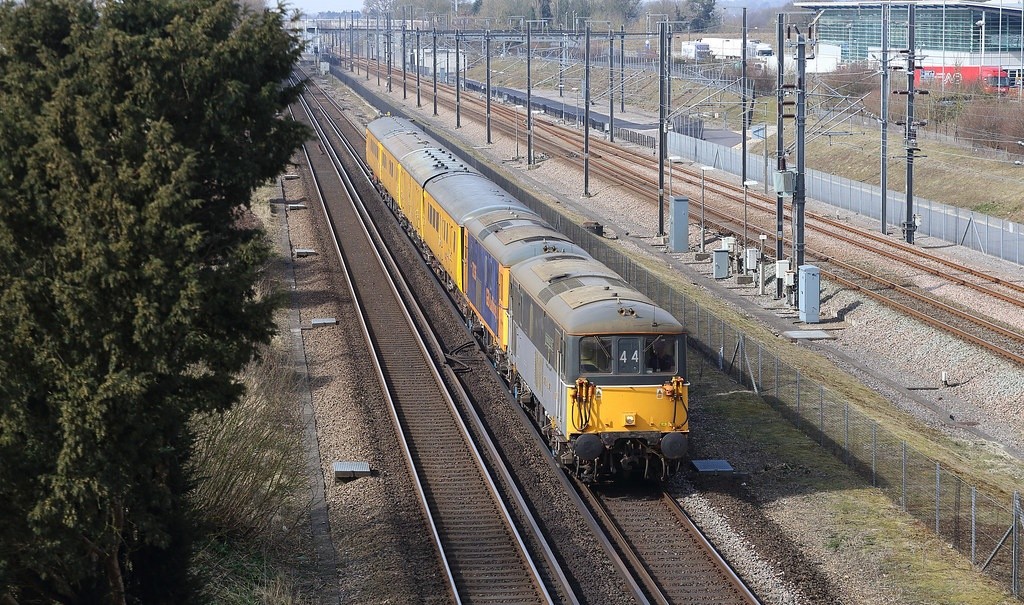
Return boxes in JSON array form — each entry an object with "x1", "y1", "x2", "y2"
[{"x1": 364, "y1": 109, "x2": 692, "y2": 491}]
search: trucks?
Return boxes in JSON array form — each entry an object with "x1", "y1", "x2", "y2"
[{"x1": 681, "y1": 41, "x2": 713, "y2": 62}]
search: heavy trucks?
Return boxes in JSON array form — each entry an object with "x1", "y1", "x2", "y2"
[
  {"x1": 912, "y1": 65, "x2": 1011, "y2": 95},
  {"x1": 699, "y1": 36, "x2": 775, "y2": 61}
]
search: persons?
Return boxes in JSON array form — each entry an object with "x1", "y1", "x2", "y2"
[{"x1": 645, "y1": 340, "x2": 675, "y2": 372}]
